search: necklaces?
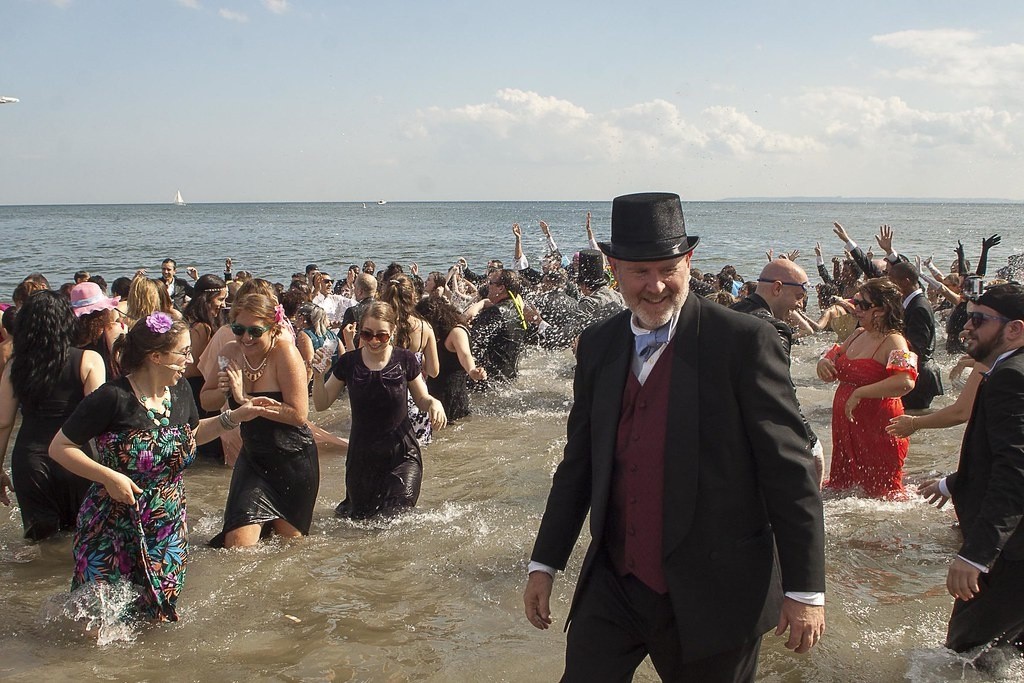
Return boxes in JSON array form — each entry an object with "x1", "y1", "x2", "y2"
[{"x1": 242, "y1": 349, "x2": 271, "y2": 383}]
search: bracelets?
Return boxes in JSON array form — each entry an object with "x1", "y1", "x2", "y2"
[
  {"x1": 219, "y1": 409, "x2": 240, "y2": 431},
  {"x1": 911, "y1": 416, "x2": 918, "y2": 430}
]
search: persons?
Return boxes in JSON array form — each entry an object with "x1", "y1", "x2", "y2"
[
  {"x1": 523, "y1": 192, "x2": 825, "y2": 683},
  {"x1": 0, "y1": 212, "x2": 1024, "y2": 552},
  {"x1": 49, "y1": 312, "x2": 281, "y2": 637},
  {"x1": 916, "y1": 283, "x2": 1024, "y2": 671}
]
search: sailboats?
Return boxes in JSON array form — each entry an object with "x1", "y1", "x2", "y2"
[{"x1": 174, "y1": 189, "x2": 187, "y2": 206}]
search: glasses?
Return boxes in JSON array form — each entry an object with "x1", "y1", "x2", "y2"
[
  {"x1": 853, "y1": 299, "x2": 879, "y2": 310},
  {"x1": 230, "y1": 320, "x2": 274, "y2": 338},
  {"x1": 321, "y1": 279, "x2": 334, "y2": 283},
  {"x1": 360, "y1": 330, "x2": 392, "y2": 343},
  {"x1": 781, "y1": 279, "x2": 809, "y2": 290},
  {"x1": 967, "y1": 310, "x2": 1011, "y2": 329},
  {"x1": 157, "y1": 347, "x2": 191, "y2": 359}
]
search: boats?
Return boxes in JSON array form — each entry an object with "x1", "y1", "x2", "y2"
[{"x1": 378, "y1": 200, "x2": 386, "y2": 205}]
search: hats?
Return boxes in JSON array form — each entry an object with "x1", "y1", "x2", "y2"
[
  {"x1": 576, "y1": 249, "x2": 611, "y2": 285},
  {"x1": 305, "y1": 264, "x2": 319, "y2": 277},
  {"x1": 598, "y1": 192, "x2": 700, "y2": 262},
  {"x1": 977, "y1": 284, "x2": 1024, "y2": 320},
  {"x1": 71, "y1": 281, "x2": 120, "y2": 316}
]
